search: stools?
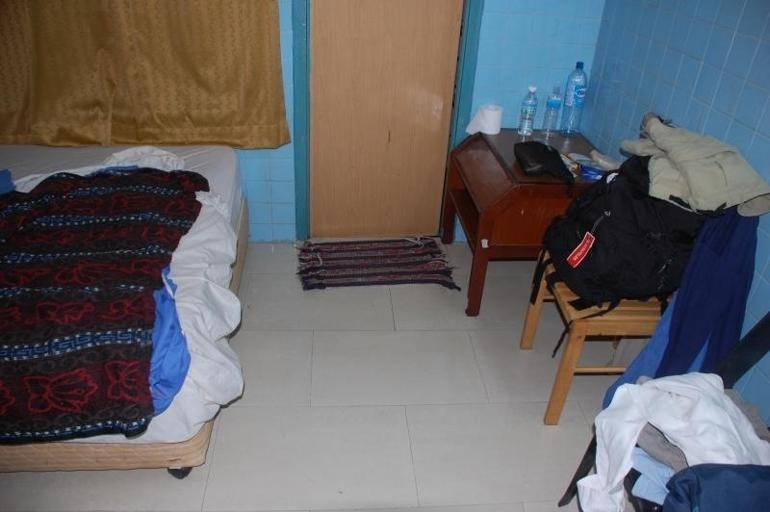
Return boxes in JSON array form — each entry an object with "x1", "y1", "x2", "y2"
[{"x1": 520, "y1": 250, "x2": 681, "y2": 426}]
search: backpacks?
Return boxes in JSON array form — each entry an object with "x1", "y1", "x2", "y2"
[{"x1": 544, "y1": 154, "x2": 708, "y2": 305}]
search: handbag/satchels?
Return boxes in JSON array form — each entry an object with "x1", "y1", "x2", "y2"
[{"x1": 514, "y1": 140, "x2": 574, "y2": 183}]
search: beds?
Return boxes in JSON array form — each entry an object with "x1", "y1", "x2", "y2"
[{"x1": 0, "y1": 143, "x2": 249, "y2": 479}]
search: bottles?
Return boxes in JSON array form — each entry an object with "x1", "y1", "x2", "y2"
[
  {"x1": 541, "y1": 87, "x2": 563, "y2": 137},
  {"x1": 561, "y1": 61, "x2": 588, "y2": 137},
  {"x1": 518, "y1": 87, "x2": 538, "y2": 137}
]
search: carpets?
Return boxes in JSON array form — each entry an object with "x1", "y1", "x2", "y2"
[{"x1": 296, "y1": 237, "x2": 460, "y2": 293}]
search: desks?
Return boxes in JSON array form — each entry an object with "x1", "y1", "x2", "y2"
[{"x1": 439, "y1": 129, "x2": 607, "y2": 317}]
